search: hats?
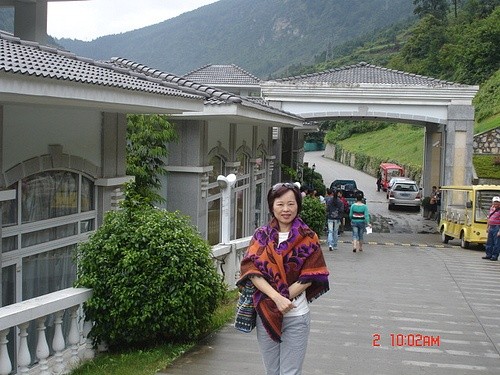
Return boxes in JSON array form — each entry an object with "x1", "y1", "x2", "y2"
[{"x1": 493, "y1": 197, "x2": 500, "y2": 201}]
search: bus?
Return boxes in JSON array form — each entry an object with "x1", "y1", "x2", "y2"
[
  {"x1": 436, "y1": 184, "x2": 500, "y2": 249},
  {"x1": 379, "y1": 162, "x2": 404, "y2": 192}
]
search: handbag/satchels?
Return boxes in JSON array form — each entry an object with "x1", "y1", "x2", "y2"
[{"x1": 233, "y1": 285, "x2": 256, "y2": 333}]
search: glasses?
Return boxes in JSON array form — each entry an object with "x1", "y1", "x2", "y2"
[{"x1": 272, "y1": 183, "x2": 296, "y2": 191}]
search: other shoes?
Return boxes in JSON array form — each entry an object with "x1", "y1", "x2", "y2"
[
  {"x1": 490, "y1": 258, "x2": 497, "y2": 261},
  {"x1": 353, "y1": 249, "x2": 356, "y2": 252},
  {"x1": 425, "y1": 218, "x2": 430, "y2": 220},
  {"x1": 482, "y1": 256, "x2": 492, "y2": 259},
  {"x1": 360, "y1": 249, "x2": 362, "y2": 251},
  {"x1": 329, "y1": 245, "x2": 333, "y2": 251}
]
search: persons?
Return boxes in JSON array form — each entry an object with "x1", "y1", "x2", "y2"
[
  {"x1": 233, "y1": 181, "x2": 331, "y2": 375},
  {"x1": 376, "y1": 167, "x2": 382, "y2": 192},
  {"x1": 482, "y1": 195, "x2": 500, "y2": 261},
  {"x1": 423, "y1": 185, "x2": 441, "y2": 225},
  {"x1": 326, "y1": 186, "x2": 345, "y2": 251},
  {"x1": 348, "y1": 190, "x2": 372, "y2": 253},
  {"x1": 293, "y1": 181, "x2": 349, "y2": 237}
]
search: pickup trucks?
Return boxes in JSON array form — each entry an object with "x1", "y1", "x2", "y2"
[{"x1": 327, "y1": 179, "x2": 366, "y2": 216}]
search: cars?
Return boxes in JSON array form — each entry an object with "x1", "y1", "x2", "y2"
[
  {"x1": 385, "y1": 177, "x2": 413, "y2": 199},
  {"x1": 387, "y1": 180, "x2": 423, "y2": 212}
]
